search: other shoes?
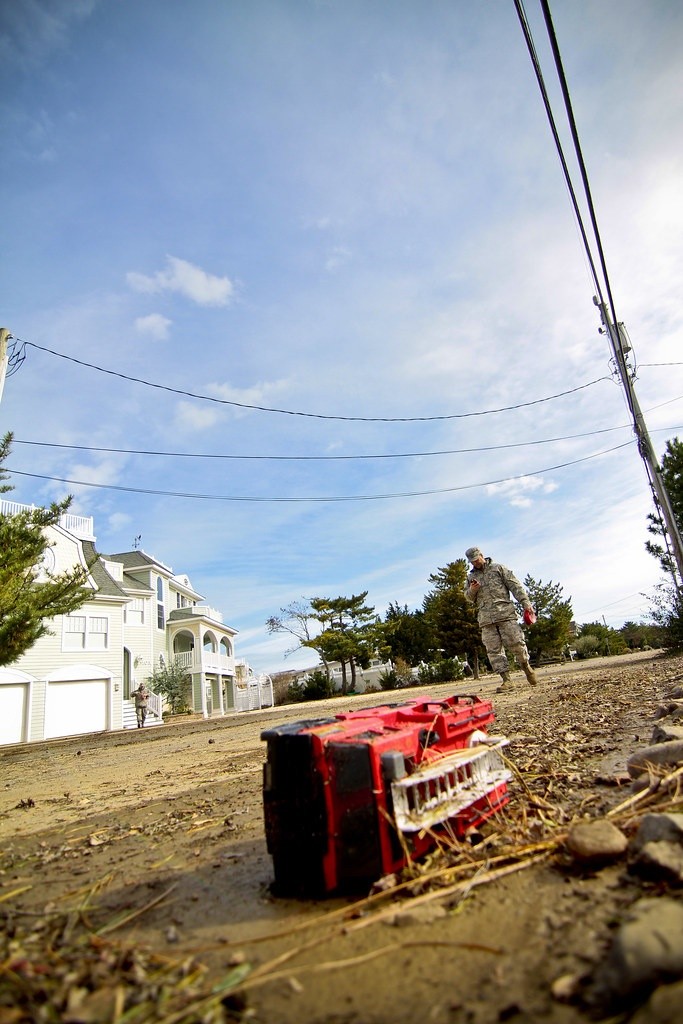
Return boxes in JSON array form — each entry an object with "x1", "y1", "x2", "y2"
[
  {"x1": 142, "y1": 722, "x2": 144, "y2": 727},
  {"x1": 521, "y1": 663, "x2": 537, "y2": 685},
  {"x1": 138, "y1": 722, "x2": 140, "y2": 729},
  {"x1": 496, "y1": 680, "x2": 516, "y2": 692}
]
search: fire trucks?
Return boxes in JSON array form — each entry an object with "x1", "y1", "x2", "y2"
[{"x1": 257, "y1": 690, "x2": 516, "y2": 903}]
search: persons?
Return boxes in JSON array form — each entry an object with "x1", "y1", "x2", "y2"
[
  {"x1": 462, "y1": 547, "x2": 539, "y2": 693},
  {"x1": 130, "y1": 683, "x2": 150, "y2": 729}
]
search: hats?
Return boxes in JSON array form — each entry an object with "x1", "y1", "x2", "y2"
[
  {"x1": 465, "y1": 545, "x2": 480, "y2": 563},
  {"x1": 139, "y1": 683, "x2": 144, "y2": 687}
]
